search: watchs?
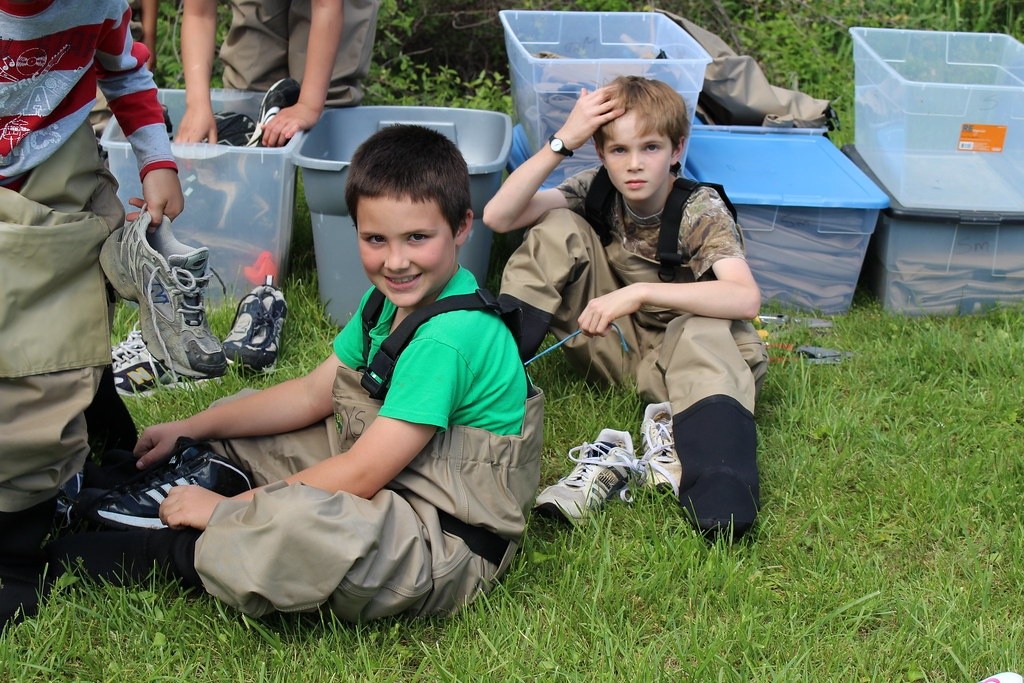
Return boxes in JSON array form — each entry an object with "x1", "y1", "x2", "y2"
[{"x1": 550, "y1": 135, "x2": 574, "y2": 158}]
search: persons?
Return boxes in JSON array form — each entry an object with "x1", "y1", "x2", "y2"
[
  {"x1": 134, "y1": 124, "x2": 545, "y2": 636},
  {"x1": 0, "y1": 0, "x2": 184, "y2": 632},
  {"x1": 176, "y1": 1, "x2": 382, "y2": 148},
  {"x1": 481, "y1": 74, "x2": 762, "y2": 541}
]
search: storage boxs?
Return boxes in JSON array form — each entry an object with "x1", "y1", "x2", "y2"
[
  {"x1": 496, "y1": 6, "x2": 1023, "y2": 320},
  {"x1": 101, "y1": 88, "x2": 304, "y2": 301},
  {"x1": 291, "y1": 107, "x2": 514, "y2": 330}
]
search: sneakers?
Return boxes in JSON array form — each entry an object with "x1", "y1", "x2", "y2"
[
  {"x1": 238, "y1": 79, "x2": 300, "y2": 183},
  {"x1": 640, "y1": 401, "x2": 684, "y2": 501},
  {"x1": 119, "y1": 105, "x2": 275, "y2": 280},
  {"x1": 224, "y1": 276, "x2": 287, "y2": 369},
  {"x1": 97, "y1": 205, "x2": 227, "y2": 378},
  {"x1": 536, "y1": 424, "x2": 638, "y2": 523},
  {"x1": 110, "y1": 321, "x2": 221, "y2": 397},
  {"x1": 86, "y1": 436, "x2": 257, "y2": 532}
]
